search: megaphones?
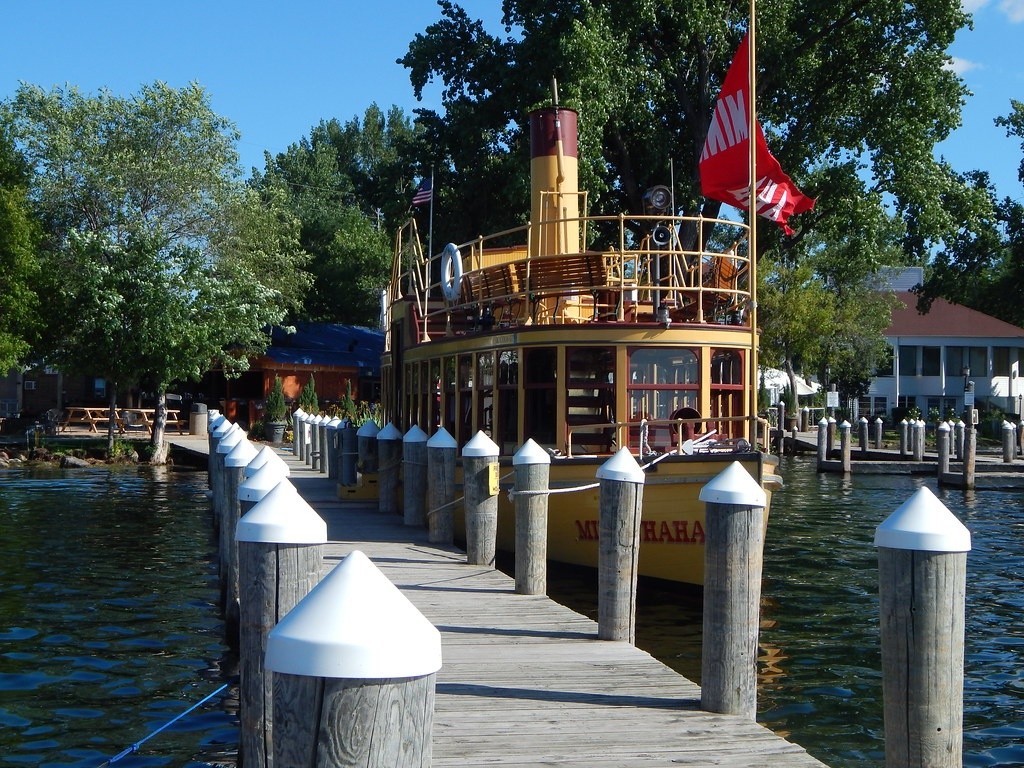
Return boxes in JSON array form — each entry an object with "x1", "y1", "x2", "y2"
[{"x1": 653, "y1": 226, "x2": 671, "y2": 245}]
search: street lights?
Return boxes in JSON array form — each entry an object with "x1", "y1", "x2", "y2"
[{"x1": 962, "y1": 365, "x2": 970, "y2": 388}]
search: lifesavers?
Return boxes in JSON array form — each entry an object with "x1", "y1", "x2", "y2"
[{"x1": 440, "y1": 242, "x2": 464, "y2": 302}]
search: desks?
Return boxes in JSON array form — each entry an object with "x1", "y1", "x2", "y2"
[
  {"x1": 62, "y1": 407, "x2": 125, "y2": 433},
  {"x1": 123, "y1": 408, "x2": 183, "y2": 435}
]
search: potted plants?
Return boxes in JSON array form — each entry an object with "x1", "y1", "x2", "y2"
[{"x1": 263, "y1": 377, "x2": 287, "y2": 443}]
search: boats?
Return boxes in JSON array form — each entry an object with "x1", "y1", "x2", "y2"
[{"x1": 369, "y1": 72, "x2": 783, "y2": 603}]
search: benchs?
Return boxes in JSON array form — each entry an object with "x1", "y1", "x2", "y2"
[
  {"x1": 677, "y1": 257, "x2": 738, "y2": 325},
  {"x1": 463, "y1": 264, "x2": 526, "y2": 331},
  {"x1": 64, "y1": 417, "x2": 125, "y2": 422},
  {"x1": 515, "y1": 255, "x2": 620, "y2": 325},
  {"x1": 143, "y1": 420, "x2": 187, "y2": 425}
]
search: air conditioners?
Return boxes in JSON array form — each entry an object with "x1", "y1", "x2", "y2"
[{"x1": 25, "y1": 381, "x2": 36, "y2": 390}]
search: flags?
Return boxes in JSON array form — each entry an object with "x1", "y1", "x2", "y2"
[
  {"x1": 412, "y1": 177, "x2": 431, "y2": 204},
  {"x1": 699, "y1": 35, "x2": 818, "y2": 236}
]
